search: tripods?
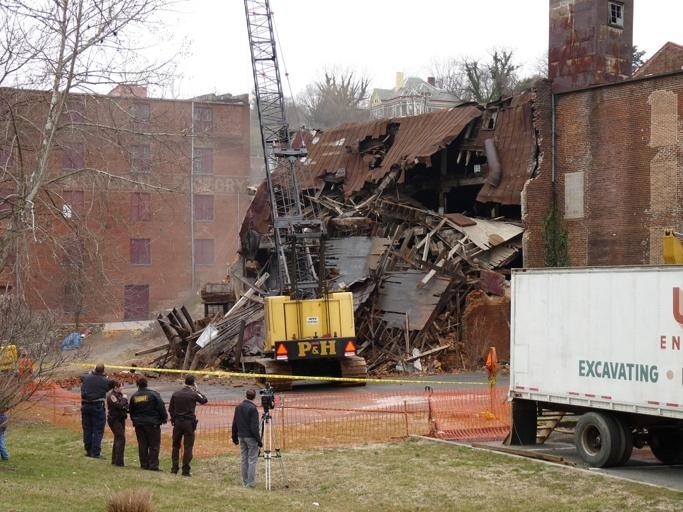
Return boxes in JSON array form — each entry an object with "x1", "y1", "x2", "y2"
[{"x1": 259, "y1": 412, "x2": 289, "y2": 491}]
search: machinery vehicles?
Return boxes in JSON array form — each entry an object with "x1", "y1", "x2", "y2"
[{"x1": 241, "y1": 0, "x2": 368, "y2": 390}]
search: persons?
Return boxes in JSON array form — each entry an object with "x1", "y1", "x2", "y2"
[
  {"x1": 106, "y1": 380, "x2": 129, "y2": 466},
  {"x1": 229, "y1": 388, "x2": 263, "y2": 487},
  {"x1": 0, "y1": 411, "x2": 9, "y2": 460},
  {"x1": 128, "y1": 378, "x2": 168, "y2": 472},
  {"x1": 79, "y1": 361, "x2": 118, "y2": 459},
  {"x1": 167, "y1": 375, "x2": 208, "y2": 477}
]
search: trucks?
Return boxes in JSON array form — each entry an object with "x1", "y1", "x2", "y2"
[{"x1": 508, "y1": 264, "x2": 682, "y2": 469}]
